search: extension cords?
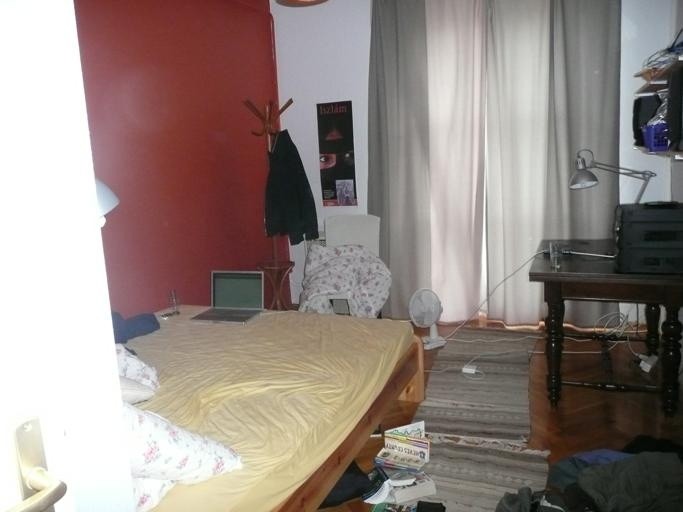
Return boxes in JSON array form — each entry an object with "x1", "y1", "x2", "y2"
[{"x1": 641, "y1": 355, "x2": 658, "y2": 373}]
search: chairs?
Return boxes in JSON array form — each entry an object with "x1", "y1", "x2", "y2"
[{"x1": 323, "y1": 214, "x2": 381, "y2": 260}]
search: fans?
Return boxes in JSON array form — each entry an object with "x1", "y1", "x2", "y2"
[{"x1": 408, "y1": 287, "x2": 444, "y2": 348}]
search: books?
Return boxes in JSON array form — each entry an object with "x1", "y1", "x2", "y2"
[{"x1": 358, "y1": 421, "x2": 437, "y2": 512}]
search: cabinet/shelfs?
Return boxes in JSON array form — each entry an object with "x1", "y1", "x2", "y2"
[
  {"x1": 632, "y1": 55, "x2": 682, "y2": 157},
  {"x1": 241, "y1": 94, "x2": 295, "y2": 310}
]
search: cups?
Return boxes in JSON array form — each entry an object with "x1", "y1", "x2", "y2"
[{"x1": 549, "y1": 241, "x2": 562, "y2": 268}]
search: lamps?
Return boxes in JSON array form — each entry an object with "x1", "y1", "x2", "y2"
[{"x1": 566, "y1": 148, "x2": 658, "y2": 230}]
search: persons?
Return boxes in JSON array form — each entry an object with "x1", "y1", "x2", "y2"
[{"x1": 319, "y1": 113, "x2": 354, "y2": 174}]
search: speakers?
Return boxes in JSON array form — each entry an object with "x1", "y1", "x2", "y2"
[{"x1": 615, "y1": 203, "x2": 682, "y2": 274}]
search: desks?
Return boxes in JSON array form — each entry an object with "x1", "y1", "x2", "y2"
[{"x1": 532, "y1": 238, "x2": 682, "y2": 407}]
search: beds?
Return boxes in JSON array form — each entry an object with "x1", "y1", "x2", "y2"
[{"x1": 105, "y1": 303, "x2": 424, "y2": 512}]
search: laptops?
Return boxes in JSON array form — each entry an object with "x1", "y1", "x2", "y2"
[{"x1": 188, "y1": 270, "x2": 265, "y2": 325}]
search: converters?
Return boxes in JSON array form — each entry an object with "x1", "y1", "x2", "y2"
[{"x1": 462, "y1": 365, "x2": 477, "y2": 374}]
